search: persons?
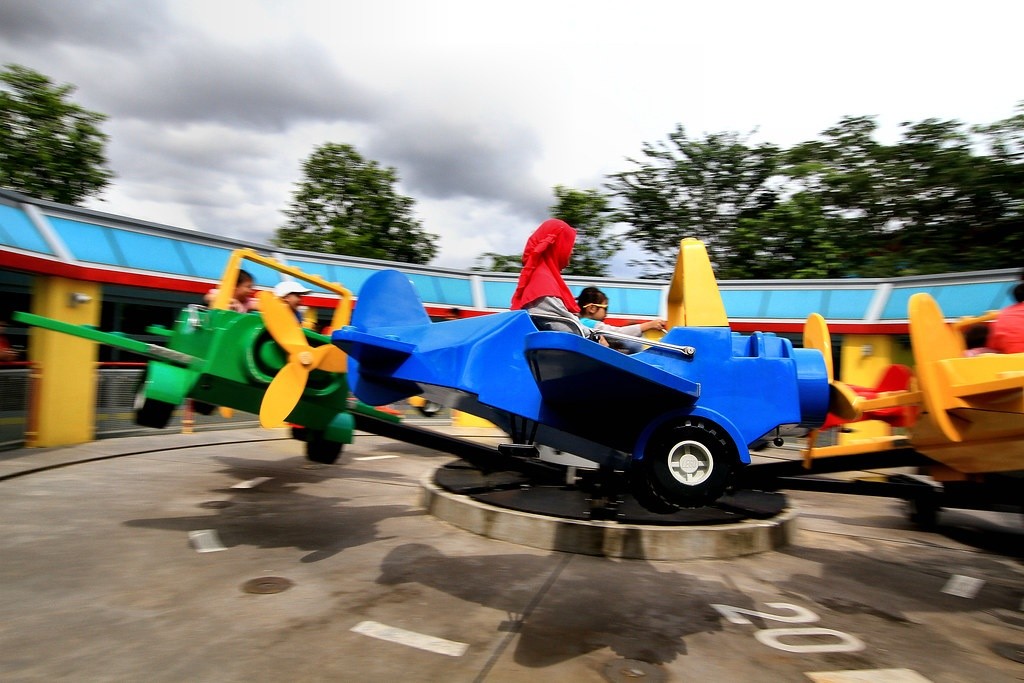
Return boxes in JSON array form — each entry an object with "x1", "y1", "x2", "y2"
[
  {"x1": 274, "y1": 281, "x2": 313, "y2": 323},
  {"x1": 204, "y1": 269, "x2": 259, "y2": 313},
  {"x1": 575, "y1": 287, "x2": 665, "y2": 339},
  {"x1": 966, "y1": 283, "x2": 1024, "y2": 357},
  {"x1": 510, "y1": 219, "x2": 609, "y2": 347},
  {"x1": 444, "y1": 308, "x2": 461, "y2": 321}
]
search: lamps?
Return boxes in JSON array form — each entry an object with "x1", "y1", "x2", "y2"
[{"x1": 67, "y1": 292, "x2": 93, "y2": 308}]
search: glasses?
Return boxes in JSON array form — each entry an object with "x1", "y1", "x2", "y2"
[{"x1": 583, "y1": 303, "x2": 609, "y2": 312}]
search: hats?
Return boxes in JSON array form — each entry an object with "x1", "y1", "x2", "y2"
[{"x1": 272, "y1": 280, "x2": 314, "y2": 297}]
